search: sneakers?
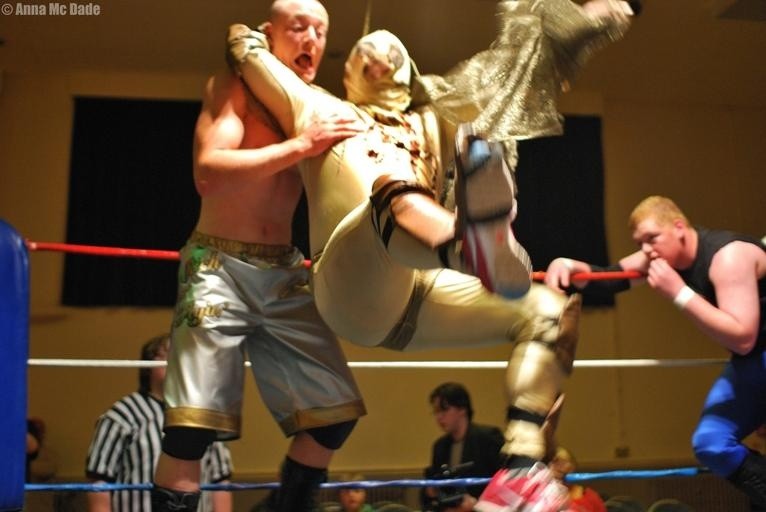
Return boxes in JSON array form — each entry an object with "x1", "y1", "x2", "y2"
[
  {"x1": 454, "y1": 136, "x2": 533, "y2": 297},
  {"x1": 475, "y1": 468, "x2": 605, "y2": 512}
]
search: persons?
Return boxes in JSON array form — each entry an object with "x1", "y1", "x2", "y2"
[
  {"x1": 83, "y1": 334, "x2": 235, "y2": 512},
  {"x1": 541, "y1": 186, "x2": 766, "y2": 511},
  {"x1": 150, "y1": 1, "x2": 371, "y2": 512},
  {"x1": 546, "y1": 441, "x2": 606, "y2": 512},
  {"x1": 426, "y1": 378, "x2": 509, "y2": 512},
  {"x1": 227, "y1": 1, "x2": 641, "y2": 512},
  {"x1": 335, "y1": 470, "x2": 375, "y2": 511}
]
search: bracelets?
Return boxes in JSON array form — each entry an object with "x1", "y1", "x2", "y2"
[
  {"x1": 672, "y1": 285, "x2": 695, "y2": 313},
  {"x1": 560, "y1": 254, "x2": 579, "y2": 273}
]
